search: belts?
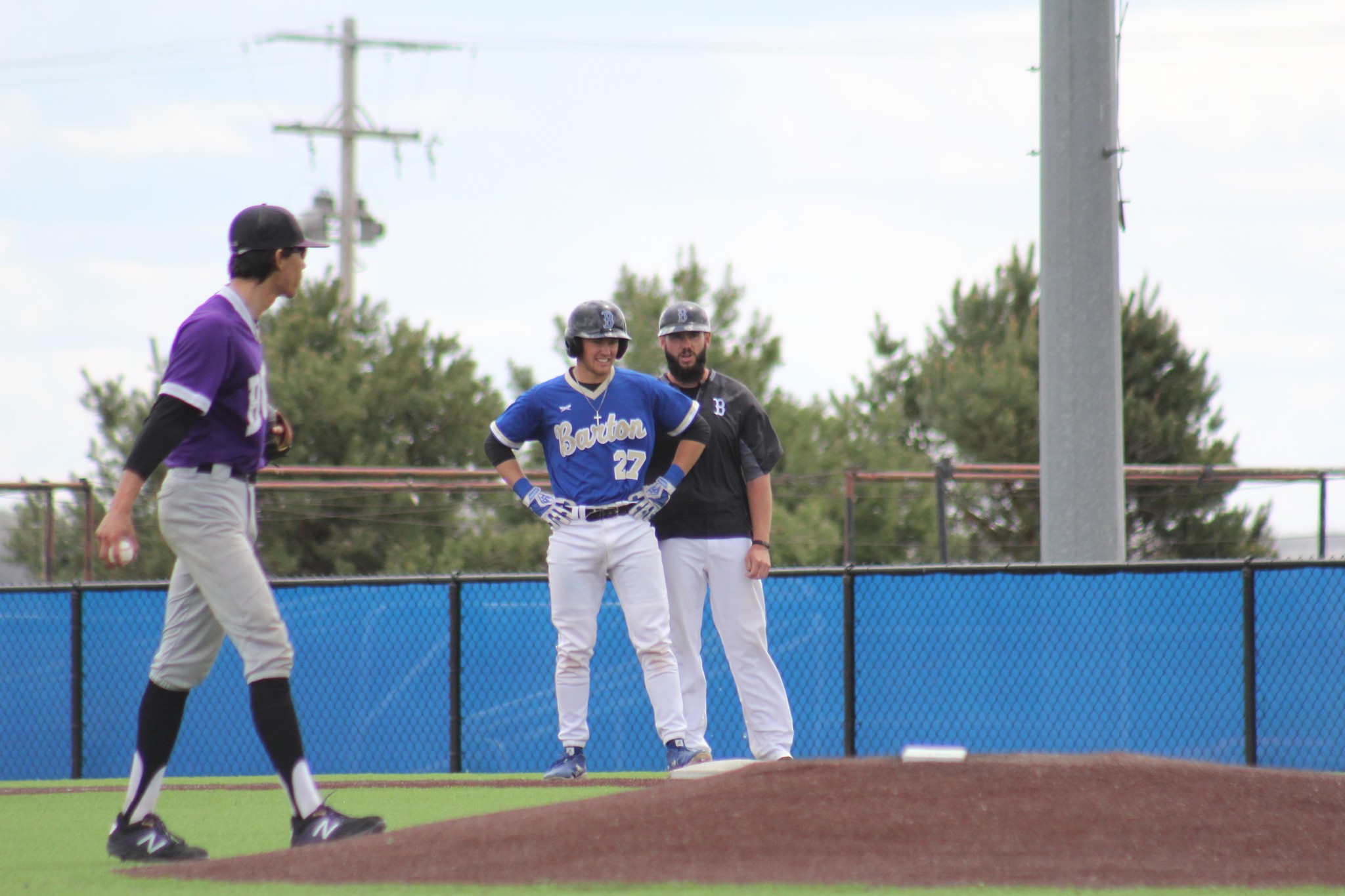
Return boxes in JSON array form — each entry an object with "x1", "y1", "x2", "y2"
[
  {"x1": 563, "y1": 502, "x2": 637, "y2": 522},
  {"x1": 196, "y1": 464, "x2": 257, "y2": 485}
]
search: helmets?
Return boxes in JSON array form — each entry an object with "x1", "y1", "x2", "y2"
[
  {"x1": 657, "y1": 300, "x2": 710, "y2": 337},
  {"x1": 565, "y1": 299, "x2": 632, "y2": 360}
]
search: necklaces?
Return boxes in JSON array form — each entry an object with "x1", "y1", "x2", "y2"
[
  {"x1": 696, "y1": 388, "x2": 701, "y2": 400},
  {"x1": 574, "y1": 368, "x2": 612, "y2": 427}
]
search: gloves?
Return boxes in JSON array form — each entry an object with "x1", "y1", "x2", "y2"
[
  {"x1": 628, "y1": 475, "x2": 676, "y2": 522},
  {"x1": 521, "y1": 485, "x2": 577, "y2": 529}
]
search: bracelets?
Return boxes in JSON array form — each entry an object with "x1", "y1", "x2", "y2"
[{"x1": 752, "y1": 540, "x2": 770, "y2": 549}]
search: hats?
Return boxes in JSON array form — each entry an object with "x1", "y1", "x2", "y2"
[{"x1": 229, "y1": 204, "x2": 330, "y2": 250}]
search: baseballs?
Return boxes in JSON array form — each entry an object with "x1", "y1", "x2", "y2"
[{"x1": 108, "y1": 539, "x2": 134, "y2": 568}]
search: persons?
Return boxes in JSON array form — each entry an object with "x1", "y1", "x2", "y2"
[
  {"x1": 92, "y1": 204, "x2": 389, "y2": 866},
  {"x1": 481, "y1": 299, "x2": 713, "y2": 781},
  {"x1": 630, "y1": 299, "x2": 796, "y2": 766}
]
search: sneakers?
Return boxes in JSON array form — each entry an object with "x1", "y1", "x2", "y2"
[
  {"x1": 666, "y1": 738, "x2": 713, "y2": 773},
  {"x1": 105, "y1": 812, "x2": 209, "y2": 862},
  {"x1": 289, "y1": 788, "x2": 387, "y2": 848},
  {"x1": 543, "y1": 753, "x2": 587, "y2": 780}
]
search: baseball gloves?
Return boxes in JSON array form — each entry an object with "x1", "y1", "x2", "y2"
[{"x1": 265, "y1": 405, "x2": 292, "y2": 460}]
